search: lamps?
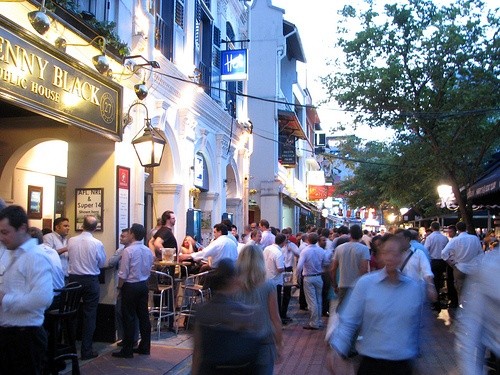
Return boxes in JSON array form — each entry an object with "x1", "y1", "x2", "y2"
[
  {"x1": 118, "y1": 103, "x2": 167, "y2": 168},
  {"x1": 26, "y1": 185, "x2": 43, "y2": 220},
  {"x1": 0, "y1": 0, "x2": 161, "y2": 101}
]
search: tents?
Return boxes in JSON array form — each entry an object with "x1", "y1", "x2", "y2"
[
  {"x1": 398, "y1": 195, "x2": 500, "y2": 234},
  {"x1": 465, "y1": 157, "x2": 500, "y2": 211}
]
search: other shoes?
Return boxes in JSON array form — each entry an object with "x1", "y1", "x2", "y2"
[
  {"x1": 112, "y1": 351, "x2": 133, "y2": 359},
  {"x1": 117, "y1": 340, "x2": 138, "y2": 347},
  {"x1": 80, "y1": 351, "x2": 98, "y2": 360},
  {"x1": 282, "y1": 317, "x2": 293, "y2": 326},
  {"x1": 132, "y1": 348, "x2": 150, "y2": 354}
]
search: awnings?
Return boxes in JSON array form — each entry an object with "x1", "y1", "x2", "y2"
[
  {"x1": 279, "y1": 193, "x2": 311, "y2": 212},
  {"x1": 297, "y1": 198, "x2": 321, "y2": 217}
]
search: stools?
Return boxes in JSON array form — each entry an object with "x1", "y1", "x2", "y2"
[
  {"x1": 146, "y1": 259, "x2": 217, "y2": 341},
  {"x1": 42, "y1": 280, "x2": 82, "y2": 375}
]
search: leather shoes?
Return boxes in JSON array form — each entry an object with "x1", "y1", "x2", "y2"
[{"x1": 303, "y1": 324, "x2": 319, "y2": 330}]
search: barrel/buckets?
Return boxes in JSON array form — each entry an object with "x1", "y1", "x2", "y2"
[{"x1": 161, "y1": 247, "x2": 176, "y2": 263}]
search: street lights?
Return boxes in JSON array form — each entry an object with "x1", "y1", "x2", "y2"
[
  {"x1": 437, "y1": 182, "x2": 452, "y2": 227},
  {"x1": 399, "y1": 206, "x2": 409, "y2": 235}
]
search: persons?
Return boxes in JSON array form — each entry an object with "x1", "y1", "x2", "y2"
[
  {"x1": 231, "y1": 245, "x2": 283, "y2": 375},
  {"x1": 0, "y1": 197, "x2": 106, "y2": 375},
  {"x1": 108, "y1": 223, "x2": 155, "y2": 358},
  {"x1": 324, "y1": 234, "x2": 431, "y2": 375},
  {"x1": 458, "y1": 246, "x2": 500, "y2": 375},
  {"x1": 149, "y1": 211, "x2": 485, "y2": 344},
  {"x1": 191, "y1": 258, "x2": 276, "y2": 375}
]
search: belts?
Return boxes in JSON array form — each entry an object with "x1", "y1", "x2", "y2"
[{"x1": 304, "y1": 274, "x2": 321, "y2": 277}]
